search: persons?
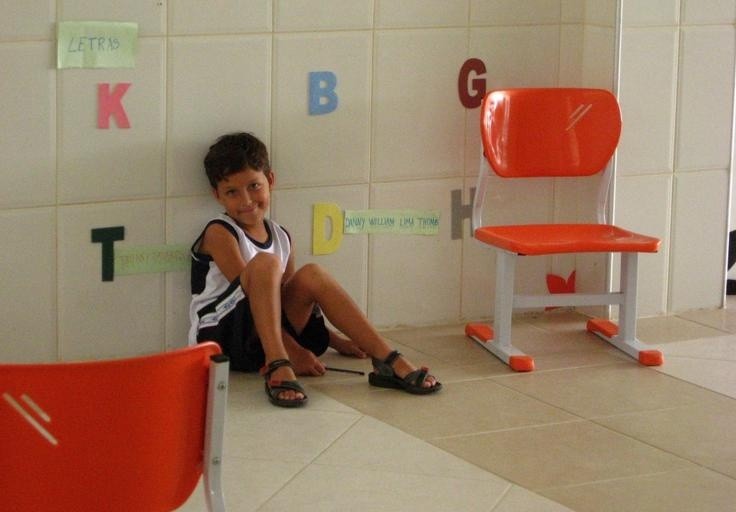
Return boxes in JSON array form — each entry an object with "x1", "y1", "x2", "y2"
[{"x1": 189, "y1": 131, "x2": 442, "y2": 409}]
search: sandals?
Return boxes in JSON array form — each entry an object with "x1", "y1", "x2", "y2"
[
  {"x1": 368, "y1": 350, "x2": 443, "y2": 394},
  {"x1": 260, "y1": 359, "x2": 308, "y2": 408}
]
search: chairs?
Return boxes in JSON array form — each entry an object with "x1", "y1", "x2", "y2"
[
  {"x1": 465, "y1": 89, "x2": 663, "y2": 372},
  {"x1": 0, "y1": 340, "x2": 230, "y2": 512}
]
